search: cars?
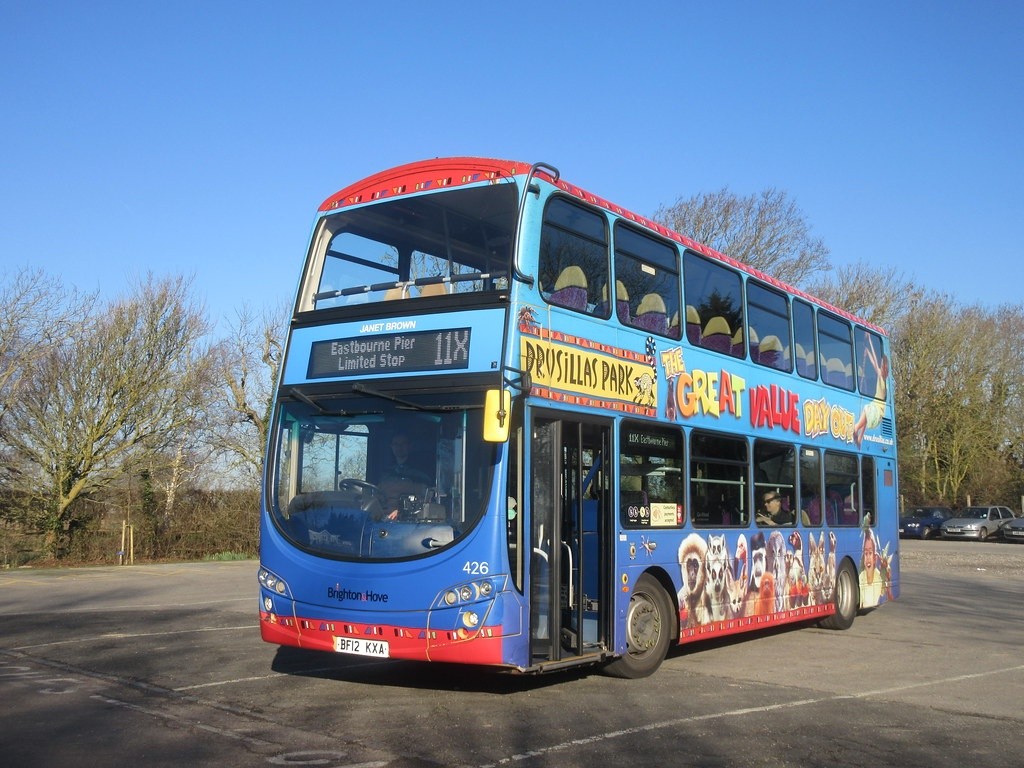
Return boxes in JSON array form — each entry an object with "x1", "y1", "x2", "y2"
[
  {"x1": 899, "y1": 506, "x2": 955, "y2": 540},
  {"x1": 938, "y1": 506, "x2": 1017, "y2": 542},
  {"x1": 1002, "y1": 511, "x2": 1024, "y2": 544}
]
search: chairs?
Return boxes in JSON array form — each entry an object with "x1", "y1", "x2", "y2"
[
  {"x1": 384, "y1": 283, "x2": 447, "y2": 301},
  {"x1": 548, "y1": 266, "x2": 869, "y2": 397},
  {"x1": 619, "y1": 489, "x2": 874, "y2": 529}
]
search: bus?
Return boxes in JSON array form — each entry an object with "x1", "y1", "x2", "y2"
[{"x1": 258, "y1": 157, "x2": 901, "y2": 678}]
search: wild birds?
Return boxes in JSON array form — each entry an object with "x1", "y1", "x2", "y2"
[{"x1": 859, "y1": 512, "x2": 873, "y2": 538}]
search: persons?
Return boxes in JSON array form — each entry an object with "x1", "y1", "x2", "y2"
[
  {"x1": 859, "y1": 529, "x2": 882, "y2": 609},
  {"x1": 756, "y1": 492, "x2": 795, "y2": 526},
  {"x1": 378, "y1": 431, "x2": 433, "y2": 520},
  {"x1": 852, "y1": 332, "x2": 890, "y2": 450}
]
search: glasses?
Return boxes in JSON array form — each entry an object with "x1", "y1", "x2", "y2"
[{"x1": 763, "y1": 495, "x2": 779, "y2": 504}]
